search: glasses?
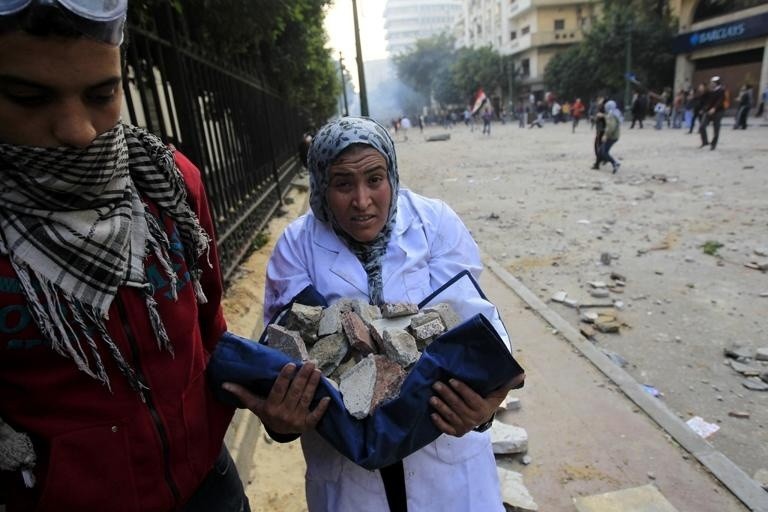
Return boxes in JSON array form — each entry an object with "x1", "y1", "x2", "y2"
[{"x1": 0, "y1": 0, "x2": 129, "y2": 22}]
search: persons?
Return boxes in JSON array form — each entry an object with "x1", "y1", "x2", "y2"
[
  {"x1": 630, "y1": 86, "x2": 704, "y2": 134},
  {"x1": 514, "y1": 92, "x2": 585, "y2": 133},
  {"x1": 589, "y1": 92, "x2": 608, "y2": 170},
  {"x1": 222, "y1": 113, "x2": 526, "y2": 512},
  {"x1": 0, "y1": 0, "x2": 253, "y2": 511},
  {"x1": 698, "y1": 75, "x2": 726, "y2": 151},
  {"x1": 595, "y1": 100, "x2": 623, "y2": 175},
  {"x1": 393, "y1": 89, "x2": 493, "y2": 134},
  {"x1": 731, "y1": 85, "x2": 766, "y2": 130}
]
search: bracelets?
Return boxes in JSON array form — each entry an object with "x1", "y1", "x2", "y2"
[{"x1": 471, "y1": 412, "x2": 496, "y2": 432}]
